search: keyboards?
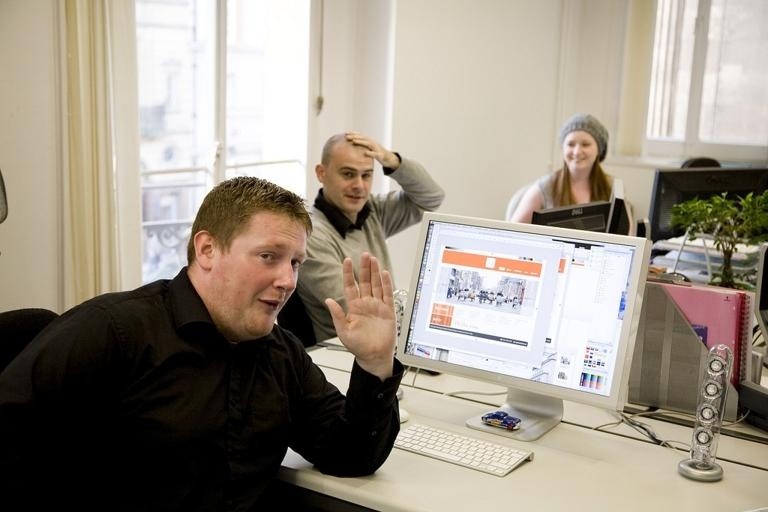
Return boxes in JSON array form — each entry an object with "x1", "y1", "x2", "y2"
[{"x1": 392, "y1": 424, "x2": 533, "y2": 477}]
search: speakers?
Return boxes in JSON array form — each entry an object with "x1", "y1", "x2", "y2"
[{"x1": 679, "y1": 344, "x2": 734, "y2": 482}]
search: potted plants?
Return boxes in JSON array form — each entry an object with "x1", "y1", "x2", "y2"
[{"x1": 670, "y1": 190, "x2": 767, "y2": 291}]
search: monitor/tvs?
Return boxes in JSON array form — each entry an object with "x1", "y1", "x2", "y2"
[
  {"x1": 605, "y1": 177, "x2": 634, "y2": 237},
  {"x1": 397, "y1": 211, "x2": 653, "y2": 441},
  {"x1": 532, "y1": 201, "x2": 611, "y2": 235},
  {"x1": 649, "y1": 168, "x2": 768, "y2": 258}
]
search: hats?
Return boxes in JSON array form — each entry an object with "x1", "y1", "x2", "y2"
[{"x1": 559, "y1": 114, "x2": 608, "y2": 156}]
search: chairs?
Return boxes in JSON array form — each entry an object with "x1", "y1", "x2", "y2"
[
  {"x1": 0, "y1": 306, "x2": 60, "y2": 374},
  {"x1": 503, "y1": 179, "x2": 530, "y2": 223},
  {"x1": 679, "y1": 156, "x2": 722, "y2": 174},
  {"x1": 275, "y1": 287, "x2": 315, "y2": 350}
]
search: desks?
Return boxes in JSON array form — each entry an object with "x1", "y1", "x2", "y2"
[
  {"x1": 301, "y1": 301, "x2": 766, "y2": 469},
  {"x1": 261, "y1": 343, "x2": 767, "y2": 512}
]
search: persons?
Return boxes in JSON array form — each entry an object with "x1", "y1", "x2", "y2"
[
  {"x1": 508, "y1": 110, "x2": 618, "y2": 224},
  {"x1": 292, "y1": 131, "x2": 446, "y2": 345},
  {"x1": 1, "y1": 176, "x2": 401, "y2": 511}
]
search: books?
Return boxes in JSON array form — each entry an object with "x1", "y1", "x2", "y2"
[
  {"x1": 650, "y1": 234, "x2": 764, "y2": 282},
  {"x1": 654, "y1": 281, "x2": 757, "y2": 387}
]
